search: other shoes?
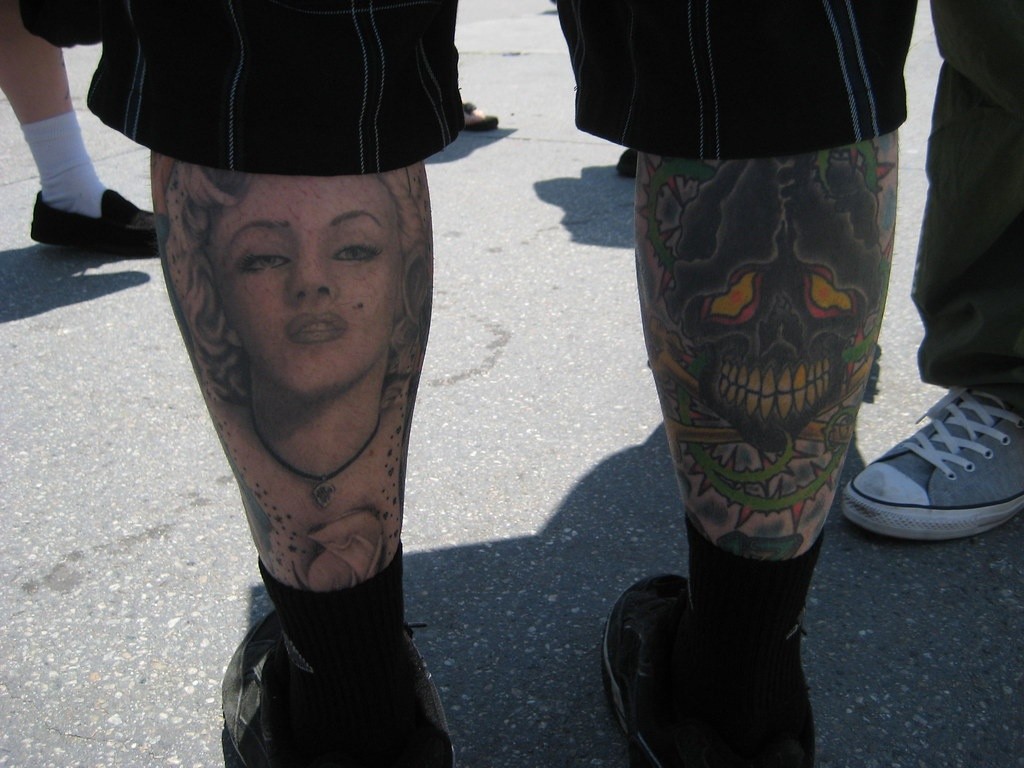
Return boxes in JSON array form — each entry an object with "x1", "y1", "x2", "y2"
[
  {"x1": 31, "y1": 189, "x2": 161, "y2": 258},
  {"x1": 601, "y1": 573, "x2": 816, "y2": 768},
  {"x1": 462, "y1": 103, "x2": 498, "y2": 131},
  {"x1": 221, "y1": 607, "x2": 452, "y2": 768},
  {"x1": 616, "y1": 149, "x2": 638, "y2": 177}
]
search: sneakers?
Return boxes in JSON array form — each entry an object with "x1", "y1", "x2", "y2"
[{"x1": 840, "y1": 385, "x2": 1024, "y2": 540}]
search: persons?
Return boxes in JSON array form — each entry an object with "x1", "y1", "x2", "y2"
[
  {"x1": 0, "y1": 0, "x2": 916, "y2": 768},
  {"x1": 838, "y1": 0, "x2": 1024, "y2": 541}
]
